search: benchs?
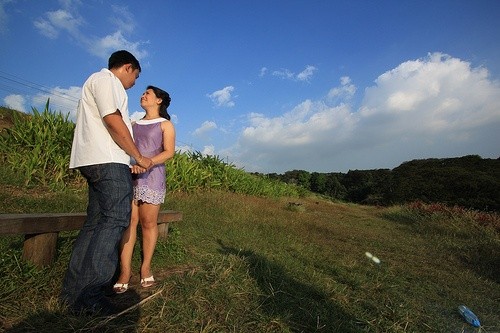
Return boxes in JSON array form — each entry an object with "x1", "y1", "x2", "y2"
[{"x1": 0, "y1": 210, "x2": 183, "y2": 265}]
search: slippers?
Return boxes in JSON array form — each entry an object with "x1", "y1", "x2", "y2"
[
  {"x1": 141, "y1": 275, "x2": 155, "y2": 288},
  {"x1": 114, "y1": 282, "x2": 129, "y2": 294}
]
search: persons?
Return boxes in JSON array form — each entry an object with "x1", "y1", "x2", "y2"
[
  {"x1": 114, "y1": 85, "x2": 175, "y2": 294},
  {"x1": 62, "y1": 50, "x2": 141, "y2": 309}
]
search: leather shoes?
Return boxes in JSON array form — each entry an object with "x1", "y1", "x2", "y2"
[{"x1": 74, "y1": 296, "x2": 129, "y2": 315}]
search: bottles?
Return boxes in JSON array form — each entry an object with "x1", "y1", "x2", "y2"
[{"x1": 457, "y1": 305, "x2": 481, "y2": 327}]
